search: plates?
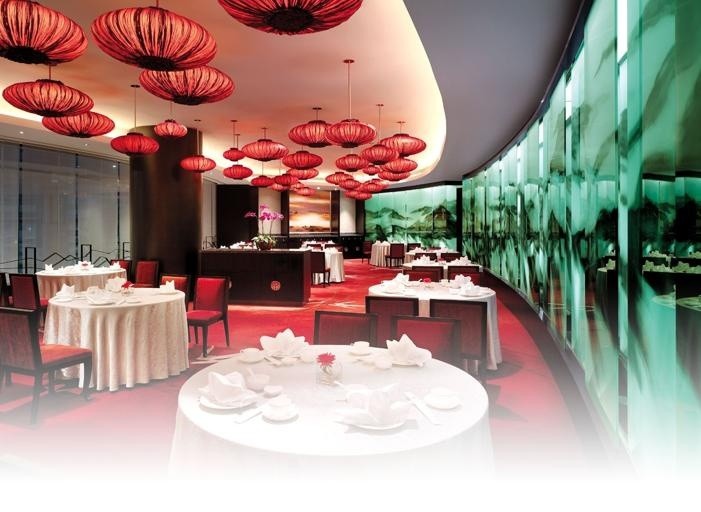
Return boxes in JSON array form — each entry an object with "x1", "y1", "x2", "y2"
[
  {"x1": 426, "y1": 386, "x2": 459, "y2": 409},
  {"x1": 264, "y1": 408, "x2": 298, "y2": 421},
  {"x1": 384, "y1": 290, "x2": 399, "y2": 294},
  {"x1": 90, "y1": 301, "x2": 113, "y2": 304},
  {"x1": 126, "y1": 298, "x2": 142, "y2": 303},
  {"x1": 392, "y1": 347, "x2": 431, "y2": 365},
  {"x1": 199, "y1": 388, "x2": 258, "y2": 410},
  {"x1": 238, "y1": 352, "x2": 264, "y2": 362},
  {"x1": 334, "y1": 410, "x2": 407, "y2": 430},
  {"x1": 461, "y1": 291, "x2": 481, "y2": 296}
]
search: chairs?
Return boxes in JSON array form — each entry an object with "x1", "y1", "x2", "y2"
[
  {"x1": 1, "y1": 259, "x2": 229, "y2": 423},
  {"x1": 308, "y1": 243, "x2": 486, "y2": 379}
]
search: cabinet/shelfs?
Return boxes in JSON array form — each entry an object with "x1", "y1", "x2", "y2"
[{"x1": 200, "y1": 244, "x2": 312, "y2": 308}]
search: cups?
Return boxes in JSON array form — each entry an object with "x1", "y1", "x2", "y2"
[
  {"x1": 247, "y1": 376, "x2": 270, "y2": 391},
  {"x1": 375, "y1": 353, "x2": 391, "y2": 369},
  {"x1": 270, "y1": 395, "x2": 290, "y2": 413},
  {"x1": 240, "y1": 348, "x2": 259, "y2": 359},
  {"x1": 451, "y1": 280, "x2": 458, "y2": 288},
  {"x1": 439, "y1": 279, "x2": 448, "y2": 287},
  {"x1": 353, "y1": 341, "x2": 369, "y2": 355}
]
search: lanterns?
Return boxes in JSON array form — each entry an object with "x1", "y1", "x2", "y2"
[
  {"x1": 216, "y1": 0, "x2": 365, "y2": 36},
  {"x1": 41, "y1": 111, "x2": 115, "y2": 138},
  {"x1": 0, "y1": 0, "x2": 89, "y2": 65},
  {"x1": 154, "y1": 119, "x2": 188, "y2": 139},
  {"x1": 178, "y1": 120, "x2": 426, "y2": 200},
  {"x1": 2, "y1": 79, "x2": 94, "y2": 117},
  {"x1": 139, "y1": 66, "x2": 235, "y2": 106},
  {"x1": 110, "y1": 132, "x2": 161, "y2": 157},
  {"x1": 89, "y1": 6, "x2": 219, "y2": 71}
]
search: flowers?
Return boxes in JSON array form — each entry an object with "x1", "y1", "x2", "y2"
[{"x1": 246, "y1": 202, "x2": 283, "y2": 244}]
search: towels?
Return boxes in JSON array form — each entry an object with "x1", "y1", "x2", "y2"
[{"x1": 204, "y1": 329, "x2": 430, "y2": 430}]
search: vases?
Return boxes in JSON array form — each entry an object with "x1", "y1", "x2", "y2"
[{"x1": 260, "y1": 240, "x2": 272, "y2": 248}]
